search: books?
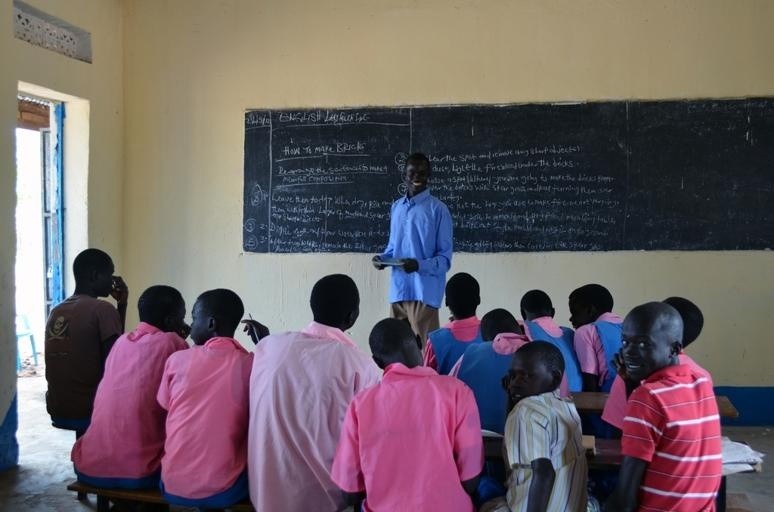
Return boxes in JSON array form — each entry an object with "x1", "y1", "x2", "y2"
[{"x1": 721, "y1": 435, "x2": 766, "y2": 478}]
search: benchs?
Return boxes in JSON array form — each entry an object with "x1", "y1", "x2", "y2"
[{"x1": 66, "y1": 482, "x2": 254, "y2": 512}]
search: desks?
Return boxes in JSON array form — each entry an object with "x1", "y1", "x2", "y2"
[
  {"x1": 569, "y1": 390, "x2": 739, "y2": 418},
  {"x1": 482, "y1": 435, "x2": 726, "y2": 512}
]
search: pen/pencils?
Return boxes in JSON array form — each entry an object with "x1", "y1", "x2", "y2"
[
  {"x1": 381, "y1": 249, "x2": 394, "y2": 257},
  {"x1": 248, "y1": 314, "x2": 260, "y2": 344}
]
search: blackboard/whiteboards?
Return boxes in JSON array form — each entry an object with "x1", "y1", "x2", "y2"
[{"x1": 243, "y1": 97, "x2": 774, "y2": 253}]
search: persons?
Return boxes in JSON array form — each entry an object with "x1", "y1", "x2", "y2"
[
  {"x1": 71, "y1": 286, "x2": 191, "y2": 511},
  {"x1": 329, "y1": 318, "x2": 485, "y2": 512},
  {"x1": 369, "y1": 151, "x2": 454, "y2": 346},
  {"x1": 45, "y1": 247, "x2": 129, "y2": 437},
  {"x1": 155, "y1": 288, "x2": 270, "y2": 511},
  {"x1": 246, "y1": 274, "x2": 380, "y2": 511},
  {"x1": 422, "y1": 272, "x2": 722, "y2": 511}
]
